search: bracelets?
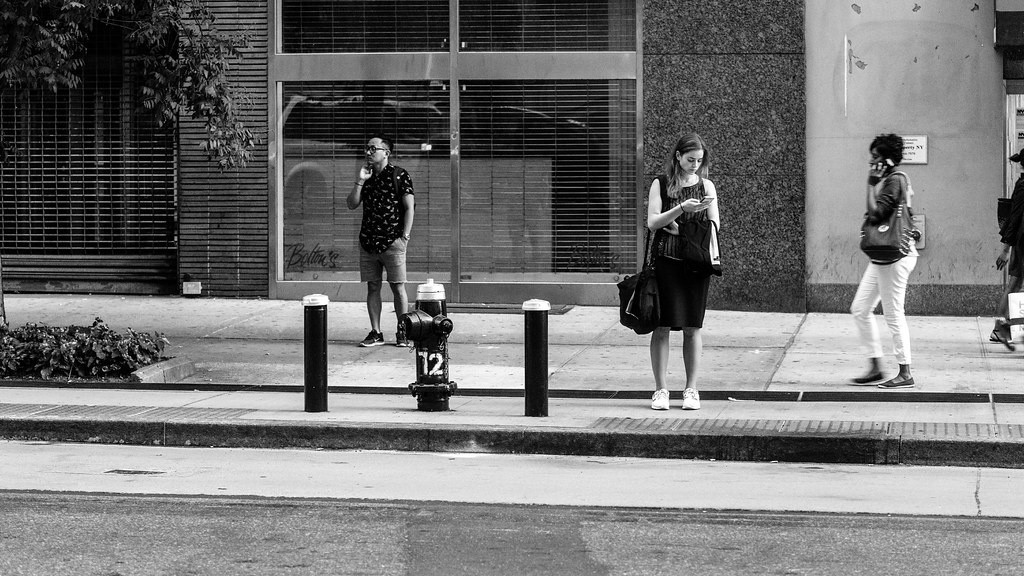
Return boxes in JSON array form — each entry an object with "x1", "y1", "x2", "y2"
[
  {"x1": 355, "y1": 183, "x2": 363, "y2": 186},
  {"x1": 404, "y1": 235, "x2": 410, "y2": 240}
]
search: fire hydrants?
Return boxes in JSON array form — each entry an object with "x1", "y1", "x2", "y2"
[{"x1": 396, "y1": 277, "x2": 457, "y2": 413}]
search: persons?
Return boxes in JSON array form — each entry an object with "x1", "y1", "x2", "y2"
[
  {"x1": 850, "y1": 132, "x2": 919, "y2": 389},
  {"x1": 647, "y1": 132, "x2": 720, "y2": 411},
  {"x1": 989, "y1": 148, "x2": 1024, "y2": 351},
  {"x1": 347, "y1": 133, "x2": 415, "y2": 347}
]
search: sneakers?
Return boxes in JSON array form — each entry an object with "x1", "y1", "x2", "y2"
[
  {"x1": 681, "y1": 387, "x2": 700, "y2": 410},
  {"x1": 652, "y1": 388, "x2": 670, "y2": 410},
  {"x1": 395, "y1": 333, "x2": 406, "y2": 347},
  {"x1": 360, "y1": 329, "x2": 385, "y2": 347}
]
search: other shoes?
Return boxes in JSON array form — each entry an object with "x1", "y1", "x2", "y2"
[
  {"x1": 849, "y1": 373, "x2": 887, "y2": 387},
  {"x1": 878, "y1": 377, "x2": 915, "y2": 388}
]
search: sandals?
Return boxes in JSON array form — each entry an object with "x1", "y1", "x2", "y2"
[{"x1": 988, "y1": 328, "x2": 1016, "y2": 353}]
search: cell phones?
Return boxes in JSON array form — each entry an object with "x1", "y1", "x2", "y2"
[
  {"x1": 878, "y1": 159, "x2": 895, "y2": 173},
  {"x1": 700, "y1": 196, "x2": 715, "y2": 204}
]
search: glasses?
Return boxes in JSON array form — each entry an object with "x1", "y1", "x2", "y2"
[{"x1": 364, "y1": 144, "x2": 388, "y2": 154}]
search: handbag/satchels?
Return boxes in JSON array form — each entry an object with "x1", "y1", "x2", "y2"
[
  {"x1": 617, "y1": 266, "x2": 664, "y2": 334},
  {"x1": 997, "y1": 197, "x2": 1011, "y2": 230},
  {"x1": 858, "y1": 175, "x2": 914, "y2": 265}
]
